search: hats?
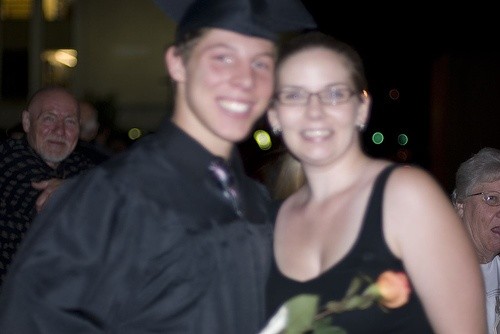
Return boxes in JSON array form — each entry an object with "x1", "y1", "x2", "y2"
[{"x1": 154, "y1": 0, "x2": 316, "y2": 42}]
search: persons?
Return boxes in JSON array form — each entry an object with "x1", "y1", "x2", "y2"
[
  {"x1": 0, "y1": 0, "x2": 280, "y2": 334},
  {"x1": 449, "y1": 147, "x2": 500, "y2": 334},
  {"x1": 0, "y1": 87, "x2": 109, "y2": 280},
  {"x1": 266, "y1": 25, "x2": 488, "y2": 334}
]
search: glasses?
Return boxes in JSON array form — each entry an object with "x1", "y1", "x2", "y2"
[
  {"x1": 467, "y1": 191, "x2": 500, "y2": 206},
  {"x1": 274, "y1": 83, "x2": 362, "y2": 107}
]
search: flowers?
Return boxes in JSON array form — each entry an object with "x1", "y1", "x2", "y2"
[{"x1": 275, "y1": 270, "x2": 410, "y2": 334}]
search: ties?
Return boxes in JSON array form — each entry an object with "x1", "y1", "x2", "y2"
[{"x1": 208, "y1": 161, "x2": 245, "y2": 223}]
